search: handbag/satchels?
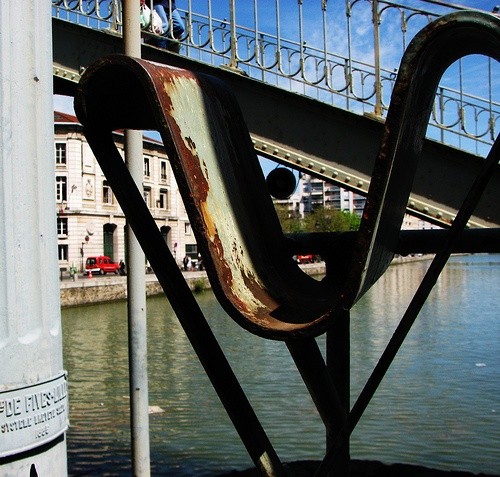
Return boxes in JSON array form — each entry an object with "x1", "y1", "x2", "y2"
[{"x1": 142, "y1": 4, "x2": 164, "y2": 34}]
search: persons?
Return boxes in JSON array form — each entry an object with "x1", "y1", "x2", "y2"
[{"x1": 146, "y1": 0, "x2": 184, "y2": 54}]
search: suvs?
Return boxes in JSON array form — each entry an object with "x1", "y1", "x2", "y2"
[{"x1": 85, "y1": 256, "x2": 118, "y2": 276}]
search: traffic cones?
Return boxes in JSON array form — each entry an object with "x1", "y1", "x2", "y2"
[{"x1": 88, "y1": 270, "x2": 92, "y2": 278}]
